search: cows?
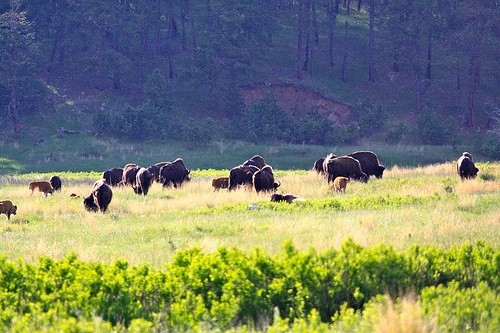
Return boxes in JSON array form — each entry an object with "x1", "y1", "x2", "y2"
[
  {"x1": 82, "y1": 179, "x2": 113, "y2": 214},
  {"x1": 28, "y1": 175, "x2": 62, "y2": 199},
  {"x1": 456, "y1": 151, "x2": 480, "y2": 182},
  {"x1": 212, "y1": 154, "x2": 281, "y2": 197},
  {"x1": 0, "y1": 199, "x2": 17, "y2": 220},
  {"x1": 269, "y1": 193, "x2": 298, "y2": 205},
  {"x1": 102, "y1": 158, "x2": 191, "y2": 197},
  {"x1": 313, "y1": 150, "x2": 386, "y2": 195}
]
